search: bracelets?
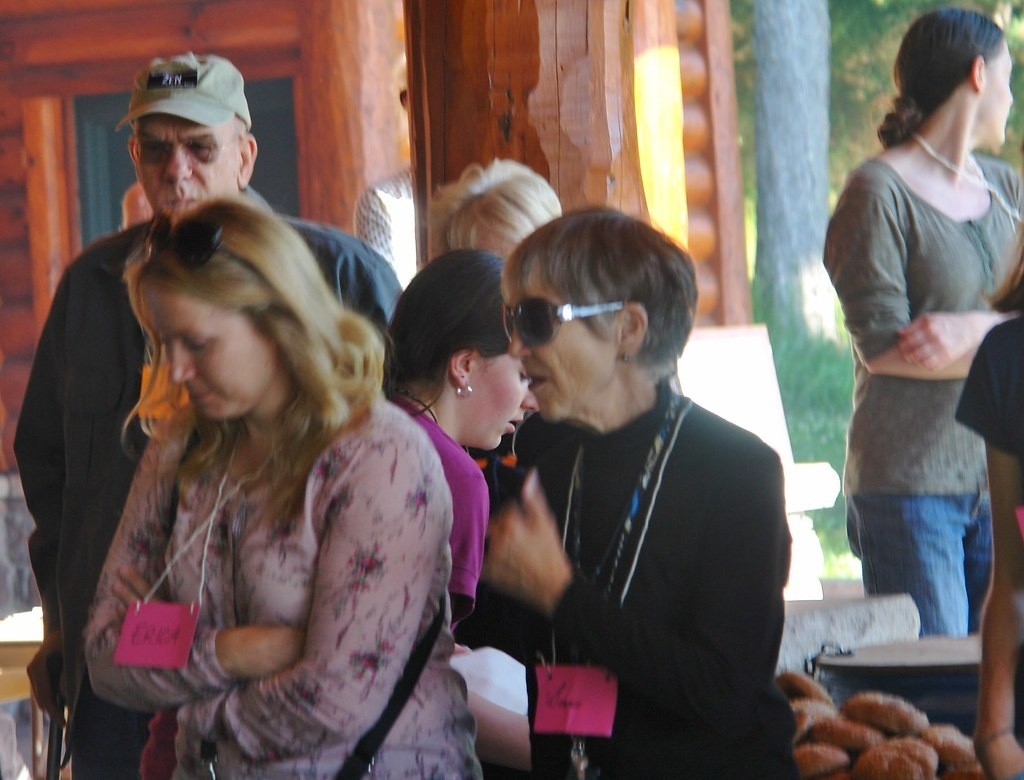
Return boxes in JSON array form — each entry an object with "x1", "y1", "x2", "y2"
[{"x1": 975, "y1": 727, "x2": 1013, "y2": 758}]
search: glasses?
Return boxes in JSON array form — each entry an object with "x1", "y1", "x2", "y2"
[
  {"x1": 145, "y1": 212, "x2": 261, "y2": 274},
  {"x1": 502, "y1": 301, "x2": 625, "y2": 344},
  {"x1": 135, "y1": 134, "x2": 242, "y2": 171}
]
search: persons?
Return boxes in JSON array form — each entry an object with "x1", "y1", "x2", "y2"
[
  {"x1": 823, "y1": 7, "x2": 1024, "y2": 638},
  {"x1": 12, "y1": 52, "x2": 484, "y2": 780},
  {"x1": 953, "y1": 224, "x2": 1024, "y2": 780},
  {"x1": 381, "y1": 156, "x2": 802, "y2": 780}
]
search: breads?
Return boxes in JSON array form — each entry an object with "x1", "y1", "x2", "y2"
[{"x1": 774, "y1": 672, "x2": 985, "y2": 780}]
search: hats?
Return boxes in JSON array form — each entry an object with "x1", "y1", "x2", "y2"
[{"x1": 115, "y1": 49, "x2": 251, "y2": 131}]
